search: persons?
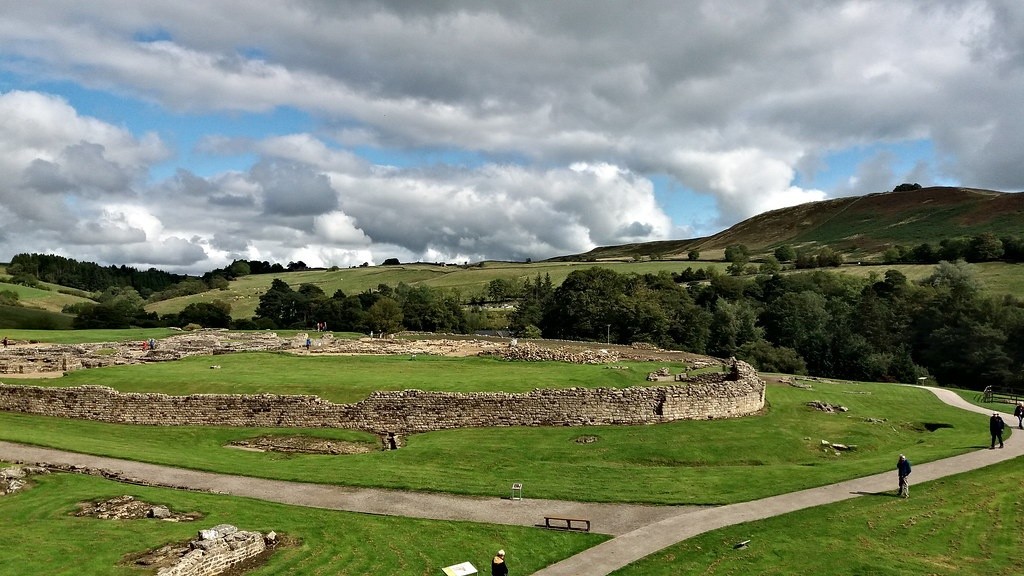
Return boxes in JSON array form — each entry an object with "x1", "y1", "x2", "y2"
[
  {"x1": 989, "y1": 411, "x2": 1004, "y2": 450},
  {"x1": 306, "y1": 339, "x2": 311, "y2": 350},
  {"x1": 4, "y1": 337, "x2": 7, "y2": 348},
  {"x1": 1014, "y1": 402, "x2": 1024, "y2": 430},
  {"x1": 897, "y1": 454, "x2": 911, "y2": 499},
  {"x1": 984, "y1": 385, "x2": 991, "y2": 403},
  {"x1": 492, "y1": 550, "x2": 508, "y2": 576},
  {"x1": 149, "y1": 339, "x2": 156, "y2": 350}
]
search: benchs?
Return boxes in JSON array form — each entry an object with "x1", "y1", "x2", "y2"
[{"x1": 544, "y1": 516, "x2": 591, "y2": 531}]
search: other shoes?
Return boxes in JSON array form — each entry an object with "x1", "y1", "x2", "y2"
[
  {"x1": 999, "y1": 446, "x2": 1004, "y2": 448},
  {"x1": 897, "y1": 493, "x2": 901, "y2": 495},
  {"x1": 990, "y1": 447, "x2": 995, "y2": 449},
  {"x1": 904, "y1": 496, "x2": 909, "y2": 499},
  {"x1": 1019, "y1": 426, "x2": 1024, "y2": 429}
]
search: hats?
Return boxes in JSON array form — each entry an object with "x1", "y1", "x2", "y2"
[
  {"x1": 1018, "y1": 402, "x2": 1023, "y2": 405},
  {"x1": 993, "y1": 411, "x2": 998, "y2": 415}
]
